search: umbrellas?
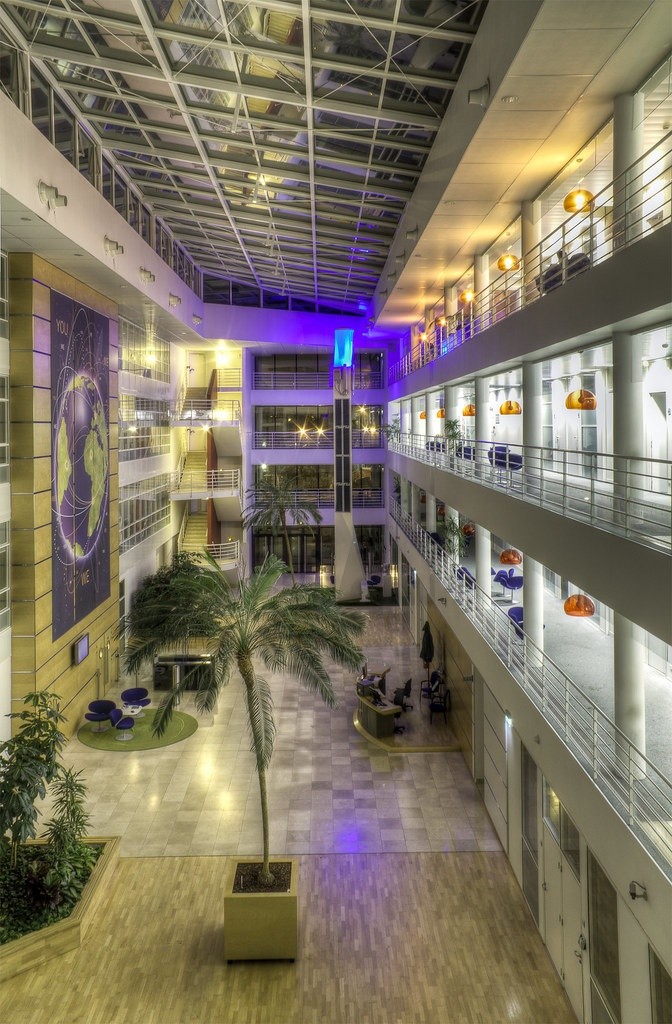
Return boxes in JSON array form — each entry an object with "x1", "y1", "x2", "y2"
[{"x1": 420, "y1": 621, "x2": 434, "y2": 696}]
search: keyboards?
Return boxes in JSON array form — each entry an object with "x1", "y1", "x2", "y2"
[
  {"x1": 380, "y1": 703, "x2": 387, "y2": 706},
  {"x1": 369, "y1": 675, "x2": 375, "y2": 681}
]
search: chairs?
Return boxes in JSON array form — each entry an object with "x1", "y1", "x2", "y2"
[
  {"x1": 426, "y1": 439, "x2": 523, "y2": 488},
  {"x1": 121, "y1": 688, "x2": 152, "y2": 718},
  {"x1": 85, "y1": 700, "x2": 116, "y2": 733},
  {"x1": 508, "y1": 607, "x2": 546, "y2": 645},
  {"x1": 456, "y1": 567, "x2": 523, "y2": 606},
  {"x1": 109, "y1": 709, "x2": 135, "y2": 741},
  {"x1": 362, "y1": 662, "x2": 451, "y2": 724}
]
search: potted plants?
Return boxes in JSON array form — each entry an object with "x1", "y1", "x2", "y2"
[{"x1": 109, "y1": 544, "x2": 373, "y2": 964}]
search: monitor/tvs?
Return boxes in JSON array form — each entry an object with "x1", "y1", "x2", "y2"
[
  {"x1": 363, "y1": 664, "x2": 367, "y2": 678},
  {"x1": 369, "y1": 686, "x2": 381, "y2": 703},
  {"x1": 74, "y1": 632, "x2": 89, "y2": 666}
]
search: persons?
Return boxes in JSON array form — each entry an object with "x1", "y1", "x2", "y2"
[{"x1": 557, "y1": 250, "x2": 566, "y2": 265}]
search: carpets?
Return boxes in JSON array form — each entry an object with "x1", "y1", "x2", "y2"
[{"x1": 77, "y1": 708, "x2": 199, "y2": 752}]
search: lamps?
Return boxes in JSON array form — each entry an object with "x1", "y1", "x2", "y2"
[
  {"x1": 564, "y1": 157, "x2": 596, "y2": 212},
  {"x1": 420, "y1": 409, "x2": 427, "y2": 420},
  {"x1": 435, "y1": 502, "x2": 446, "y2": 516},
  {"x1": 565, "y1": 350, "x2": 597, "y2": 411},
  {"x1": 463, "y1": 380, "x2": 475, "y2": 416},
  {"x1": 498, "y1": 544, "x2": 522, "y2": 566},
  {"x1": 420, "y1": 491, "x2": 427, "y2": 504},
  {"x1": 497, "y1": 232, "x2": 522, "y2": 270},
  {"x1": 499, "y1": 369, "x2": 522, "y2": 415},
  {"x1": 461, "y1": 520, "x2": 476, "y2": 535},
  {"x1": 436, "y1": 389, "x2": 446, "y2": 418},
  {"x1": 564, "y1": 588, "x2": 596, "y2": 617}
]
